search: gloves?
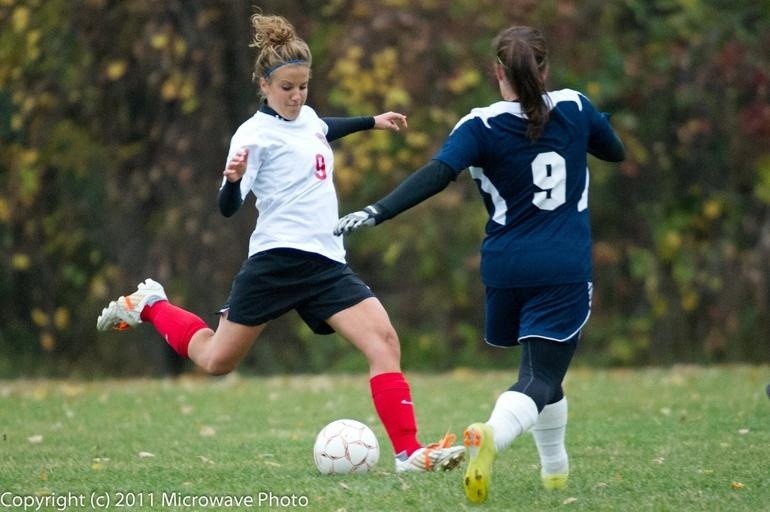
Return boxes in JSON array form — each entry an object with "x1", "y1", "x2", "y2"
[{"x1": 334, "y1": 205, "x2": 379, "y2": 236}]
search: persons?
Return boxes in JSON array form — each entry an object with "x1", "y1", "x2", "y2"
[
  {"x1": 96, "y1": 14, "x2": 466, "y2": 477},
  {"x1": 333, "y1": 25, "x2": 626, "y2": 505}
]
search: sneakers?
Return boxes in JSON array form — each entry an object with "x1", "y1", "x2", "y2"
[
  {"x1": 96, "y1": 278, "x2": 168, "y2": 331},
  {"x1": 395, "y1": 423, "x2": 497, "y2": 503},
  {"x1": 544, "y1": 475, "x2": 567, "y2": 491}
]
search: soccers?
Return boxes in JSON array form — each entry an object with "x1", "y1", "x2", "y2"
[{"x1": 313, "y1": 419, "x2": 380, "y2": 473}]
